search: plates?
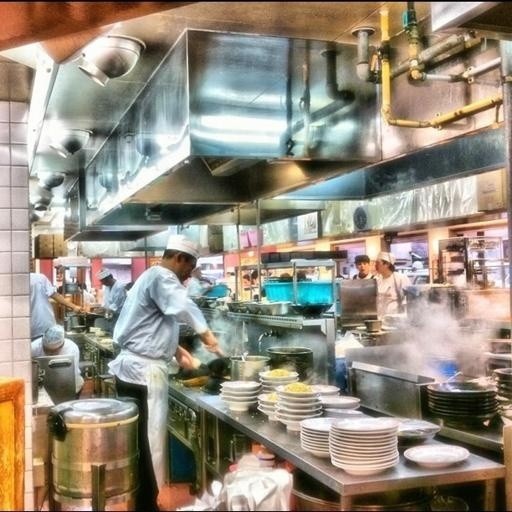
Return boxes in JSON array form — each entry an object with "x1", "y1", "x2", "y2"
[
  {"x1": 425, "y1": 380, "x2": 499, "y2": 419},
  {"x1": 492, "y1": 369, "x2": 512, "y2": 400},
  {"x1": 220, "y1": 370, "x2": 470, "y2": 476}
]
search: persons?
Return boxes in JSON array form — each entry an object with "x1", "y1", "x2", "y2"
[
  {"x1": 351, "y1": 254, "x2": 376, "y2": 281},
  {"x1": 83, "y1": 283, "x2": 96, "y2": 305},
  {"x1": 242, "y1": 274, "x2": 250, "y2": 285},
  {"x1": 29, "y1": 273, "x2": 89, "y2": 344},
  {"x1": 111, "y1": 234, "x2": 226, "y2": 512},
  {"x1": 30, "y1": 322, "x2": 88, "y2": 409},
  {"x1": 95, "y1": 267, "x2": 126, "y2": 316},
  {"x1": 187, "y1": 262, "x2": 209, "y2": 297},
  {"x1": 424, "y1": 244, "x2": 488, "y2": 310},
  {"x1": 251, "y1": 270, "x2": 264, "y2": 285},
  {"x1": 372, "y1": 251, "x2": 413, "y2": 318}
]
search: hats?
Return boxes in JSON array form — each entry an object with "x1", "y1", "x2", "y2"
[
  {"x1": 164, "y1": 234, "x2": 203, "y2": 261},
  {"x1": 376, "y1": 250, "x2": 396, "y2": 264},
  {"x1": 40, "y1": 323, "x2": 66, "y2": 352},
  {"x1": 410, "y1": 260, "x2": 424, "y2": 271},
  {"x1": 96, "y1": 268, "x2": 111, "y2": 280}
]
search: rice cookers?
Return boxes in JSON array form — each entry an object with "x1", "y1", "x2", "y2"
[{"x1": 48, "y1": 398, "x2": 138, "y2": 510}]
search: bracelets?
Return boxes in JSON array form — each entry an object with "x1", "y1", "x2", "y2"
[{"x1": 74, "y1": 306, "x2": 81, "y2": 312}]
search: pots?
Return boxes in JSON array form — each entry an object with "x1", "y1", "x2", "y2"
[{"x1": 265, "y1": 345, "x2": 314, "y2": 380}]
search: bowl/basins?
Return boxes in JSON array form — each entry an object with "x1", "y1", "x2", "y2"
[
  {"x1": 188, "y1": 292, "x2": 334, "y2": 319},
  {"x1": 262, "y1": 281, "x2": 335, "y2": 306},
  {"x1": 294, "y1": 484, "x2": 437, "y2": 511}
]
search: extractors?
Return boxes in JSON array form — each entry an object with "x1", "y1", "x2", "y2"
[{"x1": 66, "y1": 28, "x2": 387, "y2": 242}]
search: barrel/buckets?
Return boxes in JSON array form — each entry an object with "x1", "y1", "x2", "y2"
[
  {"x1": 64, "y1": 283, "x2": 79, "y2": 293},
  {"x1": 208, "y1": 284, "x2": 228, "y2": 299},
  {"x1": 265, "y1": 347, "x2": 315, "y2": 385},
  {"x1": 263, "y1": 280, "x2": 334, "y2": 306}
]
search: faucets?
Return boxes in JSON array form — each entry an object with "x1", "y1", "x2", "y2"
[{"x1": 257, "y1": 330, "x2": 280, "y2": 352}]
